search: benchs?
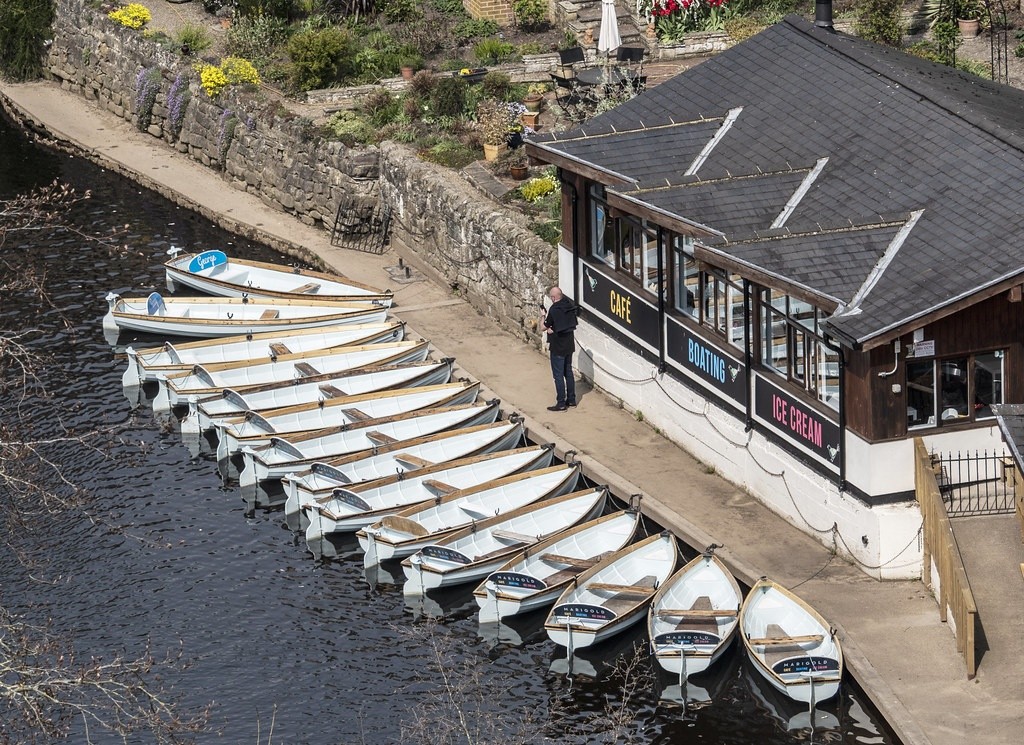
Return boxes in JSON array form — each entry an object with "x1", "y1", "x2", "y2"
[
  {"x1": 586, "y1": 582, "x2": 654, "y2": 596},
  {"x1": 260, "y1": 309, "x2": 279, "y2": 319},
  {"x1": 208, "y1": 269, "x2": 250, "y2": 284},
  {"x1": 393, "y1": 452, "x2": 434, "y2": 467},
  {"x1": 341, "y1": 408, "x2": 372, "y2": 421},
  {"x1": 269, "y1": 342, "x2": 292, "y2": 355},
  {"x1": 181, "y1": 354, "x2": 200, "y2": 363},
  {"x1": 289, "y1": 282, "x2": 320, "y2": 293},
  {"x1": 492, "y1": 529, "x2": 542, "y2": 544},
  {"x1": 319, "y1": 384, "x2": 348, "y2": 398},
  {"x1": 635, "y1": 263, "x2": 656, "y2": 272},
  {"x1": 749, "y1": 634, "x2": 824, "y2": 646},
  {"x1": 539, "y1": 553, "x2": 597, "y2": 568},
  {"x1": 657, "y1": 609, "x2": 738, "y2": 617},
  {"x1": 459, "y1": 503, "x2": 496, "y2": 517},
  {"x1": 294, "y1": 362, "x2": 322, "y2": 377},
  {"x1": 164, "y1": 306, "x2": 190, "y2": 316},
  {"x1": 422, "y1": 479, "x2": 459, "y2": 494},
  {"x1": 365, "y1": 431, "x2": 399, "y2": 444}
]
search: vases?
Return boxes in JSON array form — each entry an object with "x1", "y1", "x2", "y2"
[
  {"x1": 518, "y1": 111, "x2": 540, "y2": 132},
  {"x1": 451, "y1": 68, "x2": 489, "y2": 84}
]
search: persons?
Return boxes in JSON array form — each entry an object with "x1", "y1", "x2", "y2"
[{"x1": 540, "y1": 287, "x2": 578, "y2": 412}]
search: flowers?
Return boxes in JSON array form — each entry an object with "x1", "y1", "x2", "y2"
[{"x1": 457, "y1": 68, "x2": 477, "y2": 75}]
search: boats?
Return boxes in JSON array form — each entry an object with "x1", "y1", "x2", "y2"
[
  {"x1": 648, "y1": 549, "x2": 743, "y2": 687},
  {"x1": 544, "y1": 529, "x2": 678, "y2": 662},
  {"x1": 281, "y1": 417, "x2": 525, "y2": 515},
  {"x1": 239, "y1": 398, "x2": 501, "y2": 487},
  {"x1": 103, "y1": 292, "x2": 390, "y2": 338},
  {"x1": 164, "y1": 245, "x2": 394, "y2": 308},
  {"x1": 213, "y1": 380, "x2": 481, "y2": 462},
  {"x1": 121, "y1": 320, "x2": 408, "y2": 388},
  {"x1": 302, "y1": 443, "x2": 556, "y2": 541},
  {"x1": 152, "y1": 339, "x2": 432, "y2": 412},
  {"x1": 740, "y1": 575, "x2": 843, "y2": 714},
  {"x1": 355, "y1": 461, "x2": 582, "y2": 569},
  {"x1": 604, "y1": 234, "x2": 840, "y2": 412},
  {"x1": 400, "y1": 484, "x2": 609, "y2": 595},
  {"x1": 471, "y1": 505, "x2": 642, "y2": 623},
  {"x1": 180, "y1": 357, "x2": 456, "y2": 434}
]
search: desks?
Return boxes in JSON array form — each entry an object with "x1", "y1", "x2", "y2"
[
  {"x1": 575, "y1": 69, "x2": 622, "y2": 109},
  {"x1": 973, "y1": 355, "x2": 1001, "y2": 403}
]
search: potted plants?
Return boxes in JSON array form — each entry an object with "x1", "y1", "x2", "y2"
[
  {"x1": 398, "y1": 54, "x2": 423, "y2": 80},
  {"x1": 464, "y1": 100, "x2": 516, "y2": 162},
  {"x1": 921, "y1": 0, "x2": 987, "y2": 39},
  {"x1": 522, "y1": 83, "x2": 549, "y2": 113},
  {"x1": 482, "y1": 144, "x2": 529, "y2": 181}
]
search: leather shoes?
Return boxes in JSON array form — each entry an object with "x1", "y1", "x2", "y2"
[
  {"x1": 547, "y1": 404, "x2": 567, "y2": 412},
  {"x1": 566, "y1": 400, "x2": 576, "y2": 407}
]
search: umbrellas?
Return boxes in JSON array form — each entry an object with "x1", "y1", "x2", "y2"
[{"x1": 598, "y1": 0, "x2": 622, "y2": 75}]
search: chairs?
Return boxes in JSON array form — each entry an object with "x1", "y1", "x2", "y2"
[
  {"x1": 616, "y1": 47, "x2": 645, "y2": 77},
  {"x1": 618, "y1": 75, "x2": 648, "y2": 103},
  {"x1": 556, "y1": 47, "x2": 595, "y2": 100},
  {"x1": 548, "y1": 73, "x2": 586, "y2": 129}
]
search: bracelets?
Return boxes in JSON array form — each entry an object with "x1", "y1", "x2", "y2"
[{"x1": 542, "y1": 315, "x2": 545, "y2": 317}]
